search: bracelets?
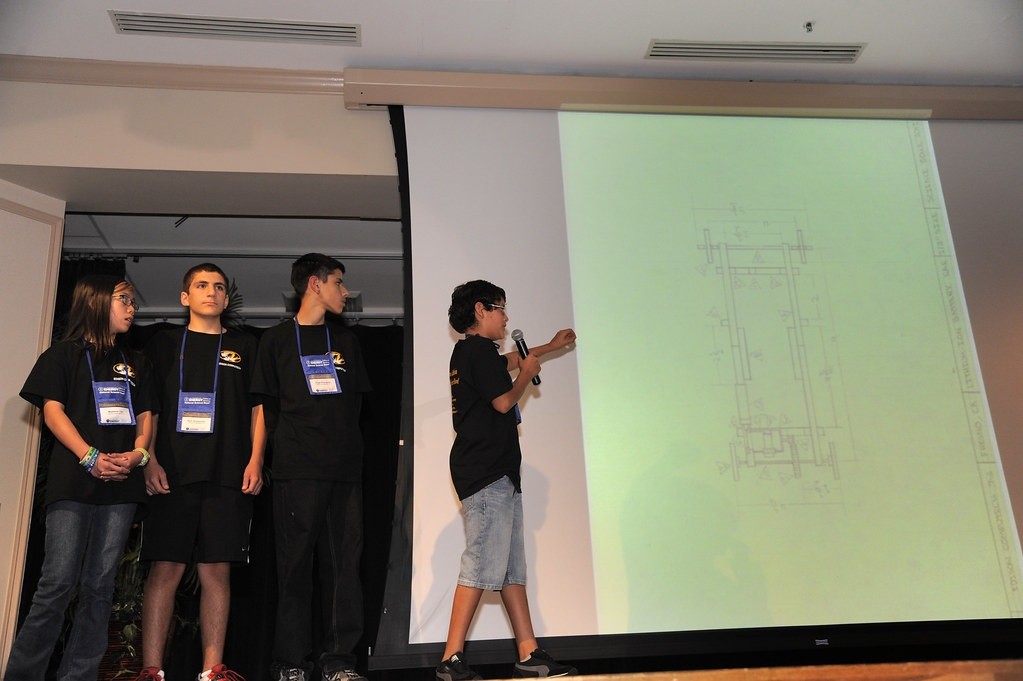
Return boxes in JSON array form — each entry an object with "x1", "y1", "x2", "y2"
[{"x1": 79, "y1": 446, "x2": 99, "y2": 472}]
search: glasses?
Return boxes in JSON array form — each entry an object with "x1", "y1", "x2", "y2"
[
  {"x1": 487, "y1": 303, "x2": 510, "y2": 313},
  {"x1": 113, "y1": 295, "x2": 139, "y2": 311}
]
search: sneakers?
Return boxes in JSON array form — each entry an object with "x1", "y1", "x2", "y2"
[
  {"x1": 134, "y1": 667, "x2": 164, "y2": 681},
  {"x1": 321, "y1": 668, "x2": 367, "y2": 681},
  {"x1": 278, "y1": 667, "x2": 306, "y2": 681},
  {"x1": 435, "y1": 650, "x2": 483, "y2": 681},
  {"x1": 513, "y1": 648, "x2": 578, "y2": 678},
  {"x1": 194, "y1": 663, "x2": 244, "y2": 681}
]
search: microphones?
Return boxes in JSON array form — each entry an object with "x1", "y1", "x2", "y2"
[{"x1": 510, "y1": 329, "x2": 541, "y2": 386}]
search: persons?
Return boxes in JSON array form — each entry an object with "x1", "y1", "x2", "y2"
[
  {"x1": 435, "y1": 279, "x2": 577, "y2": 681},
  {"x1": 132, "y1": 263, "x2": 270, "y2": 681},
  {"x1": 249, "y1": 253, "x2": 374, "y2": 681},
  {"x1": 3, "y1": 266, "x2": 155, "y2": 681}
]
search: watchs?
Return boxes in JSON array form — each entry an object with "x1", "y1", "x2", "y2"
[{"x1": 133, "y1": 447, "x2": 150, "y2": 467}]
